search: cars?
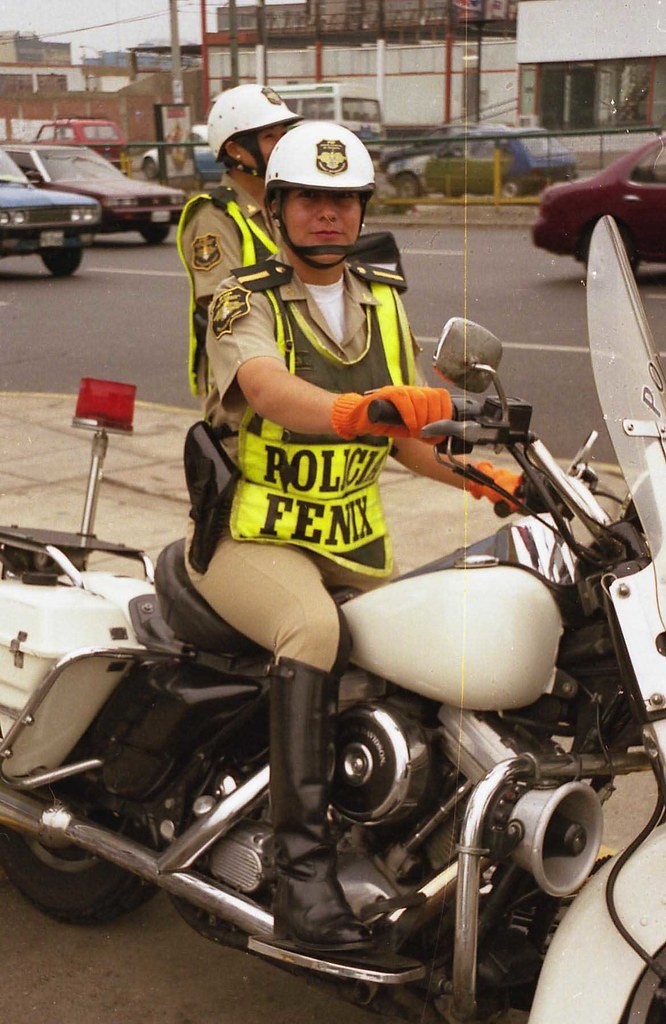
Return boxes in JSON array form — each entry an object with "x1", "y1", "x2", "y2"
[
  {"x1": 376, "y1": 121, "x2": 579, "y2": 200},
  {"x1": 531, "y1": 134, "x2": 666, "y2": 281},
  {"x1": 140, "y1": 124, "x2": 229, "y2": 190},
  {"x1": 0, "y1": 149, "x2": 101, "y2": 279},
  {"x1": 2, "y1": 142, "x2": 189, "y2": 248}
]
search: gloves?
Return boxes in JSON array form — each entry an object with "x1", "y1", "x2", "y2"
[
  {"x1": 468, "y1": 462, "x2": 526, "y2": 512},
  {"x1": 333, "y1": 386, "x2": 453, "y2": 445}
]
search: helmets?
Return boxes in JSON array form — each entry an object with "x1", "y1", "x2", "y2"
[
  {"x1": 208, "y1": 84, "x2": 305, "y2": 163},
  {"x1": 265, "y1": 122, "x2": 376, "y2": 205}
]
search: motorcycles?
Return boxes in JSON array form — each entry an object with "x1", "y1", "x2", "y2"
[{"x1": 0, "y1": 213, "x2": 664, "y2": 1024}]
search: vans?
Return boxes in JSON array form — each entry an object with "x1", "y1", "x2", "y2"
[{"x1": 33, "y1": 118, "x2": 129, "y2": 176}]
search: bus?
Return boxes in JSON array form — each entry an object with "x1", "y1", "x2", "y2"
[{"x1": 266, "y1": 83, "x2": 384, "y2": 143}]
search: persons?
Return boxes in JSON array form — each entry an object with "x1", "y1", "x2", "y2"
[
  {"x1": 185, "y1": 121, "x2": 575, "y2": 950},
  {"x1": 176, "y1": 83, "x2": 302, "y2": 403}
]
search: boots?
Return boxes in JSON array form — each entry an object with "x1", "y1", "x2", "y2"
[{"x1": 265, "y1": 656, "x2": 373, "y2": 950}]
